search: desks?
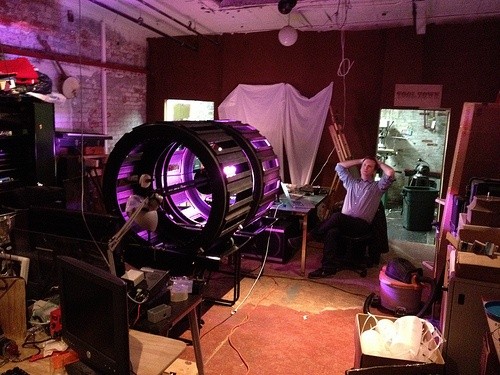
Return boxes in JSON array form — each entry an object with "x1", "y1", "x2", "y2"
[
  {"x1": 123, "y1": 262, "x2": 205, "y2": 375},
  {"x1": 0, "y1": 330, "x2": 186, "y2": 375},
  {"x1": 270, "y1": 192, "x2": 328, "y2": 276}
]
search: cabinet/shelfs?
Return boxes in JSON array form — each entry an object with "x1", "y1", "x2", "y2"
[
  {"x1": 439, "y1": 236, "x2": 500, "y2": 375},
  {"x1": 0, "y1": 94, "x2": 56, "y2": 212}
]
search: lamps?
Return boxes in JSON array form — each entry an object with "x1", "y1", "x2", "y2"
[
  {"x1": 278, "y1": 0, "x2": 297, "y2": 46},
  {"x1": 107, "y1": 189, "x2": 165, "y2": 278}
]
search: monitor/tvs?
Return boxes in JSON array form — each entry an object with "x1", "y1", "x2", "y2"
[{"x1": 55, "y1": 255, "x2": 132, "y2": 375}]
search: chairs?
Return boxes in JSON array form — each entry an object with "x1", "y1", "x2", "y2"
[{"x1": 322, "y1": 200, "x2": 383, "y2": 277}]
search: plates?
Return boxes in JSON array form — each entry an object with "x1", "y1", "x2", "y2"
[{"x1": 484, "y1": 300, "x2": 500, "y2": 322}]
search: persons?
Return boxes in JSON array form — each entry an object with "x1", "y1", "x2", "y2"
[{"x1": 308, "y1": 157, "x2": 395, "y2": 279}]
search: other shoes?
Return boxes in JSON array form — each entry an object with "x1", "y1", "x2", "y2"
[
  {"x1": 288, "y1": 236, "x2": 304, "y2": 248},
  {"x1": 308, "y1": 268, "x2": 336, "y2": 279}
]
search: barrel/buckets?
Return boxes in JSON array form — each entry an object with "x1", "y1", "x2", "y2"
[
  {"x1": 379, "y1": 270, "x2": 422, "y2": 314},
  {"x1": 400, "y1": 186, "x2": 439, "y2": 233}
]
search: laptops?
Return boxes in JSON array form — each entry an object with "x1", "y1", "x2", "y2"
[{"x1": 281, "y1": 182, "x2": 315, "y2": 209}]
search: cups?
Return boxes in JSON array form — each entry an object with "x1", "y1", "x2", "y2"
[{"x1": 485, "y1": 241, "x2": 499, "y2": 256}]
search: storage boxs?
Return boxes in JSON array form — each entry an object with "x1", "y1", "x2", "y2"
[{"x1": 354, "y1": 313, "x2": 445, "y2": 375}]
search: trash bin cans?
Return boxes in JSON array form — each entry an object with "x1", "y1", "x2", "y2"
[{"x1": 401, "y1": 186, "x2": 440, "y2": 232}]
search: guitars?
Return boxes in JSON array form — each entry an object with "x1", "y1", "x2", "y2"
[{"x1": 37, "y1": 35, "x2": 79, "y2": 98}]
